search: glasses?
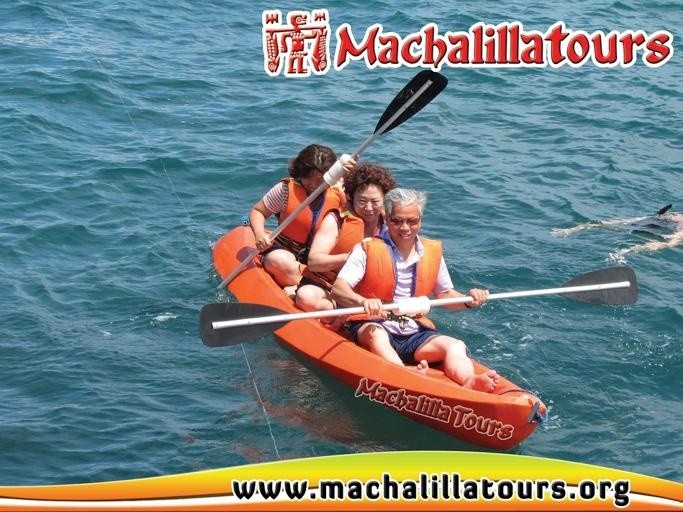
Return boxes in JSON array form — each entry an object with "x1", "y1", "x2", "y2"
[{"x1": 390, "y1": 216, "x2": 420, "y2": 226}]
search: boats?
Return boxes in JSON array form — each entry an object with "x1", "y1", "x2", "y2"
[
  {"x1": 212, "y1": 217, "x2": 548, "y2": 454},
  {"x1": 243, "y1": 340, "x2": 386, "y2": 451}
]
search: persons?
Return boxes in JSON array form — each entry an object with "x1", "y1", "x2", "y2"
[
  {"x1": 294, "y1": 161, "x2": 396, "y2": 325},
  {"x1": 248, "y1": 144, "x2": 360, "y2": 292},
  {"x1": 331, "y1": 188, "x2": 501, "y2": 393}
]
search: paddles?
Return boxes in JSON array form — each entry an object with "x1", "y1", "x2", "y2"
[
  {"x1": 218, "y1": 69, "x2": 449, "y2": 290},
  {"x1": 199, "y1": 267, "x2": 639, "y2": 347}
]
